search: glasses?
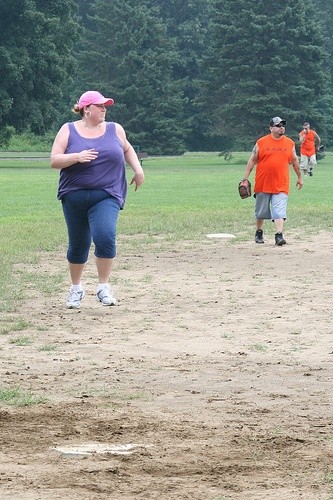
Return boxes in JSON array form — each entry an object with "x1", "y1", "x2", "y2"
[{"x1": 275, "y1": 124, "x2": 285, "y2": 127}]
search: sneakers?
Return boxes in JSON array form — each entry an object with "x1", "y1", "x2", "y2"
[
  {"x1": 95, "y1": 283, "x2": 116, "y2": 305},
  {"x1": 65, "y1": 285, "x2": 84, "y2": 309}
]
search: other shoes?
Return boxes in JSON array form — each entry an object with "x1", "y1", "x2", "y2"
[
  {"x1": 254, "y1": 229, "x2": 264, "y2": 243},
  {"x1": 275, "y1": 233, "x2": 286, "y2": 246}
]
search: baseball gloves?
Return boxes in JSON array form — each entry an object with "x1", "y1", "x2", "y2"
[{"x1": 238, "y1": 179, "x2": 252, "y2": 200}]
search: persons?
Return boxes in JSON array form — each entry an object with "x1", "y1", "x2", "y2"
[
  {"x1": 242, "y1": 117, "x2": 304, "y2": 247},
  {"x1": 50, "y1": 90, "x2": 144, "y2": 309},
  {"x1": 299, "y1": 122, "x2": 321, "y2": 177}
]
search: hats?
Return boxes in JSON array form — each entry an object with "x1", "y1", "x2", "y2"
[
  {"x1": 269, "y1": 117, "x2": 286, "y2": 126},
  {"x1": 78, "y1": 91, "x2": 114, "y2": 110}
]
